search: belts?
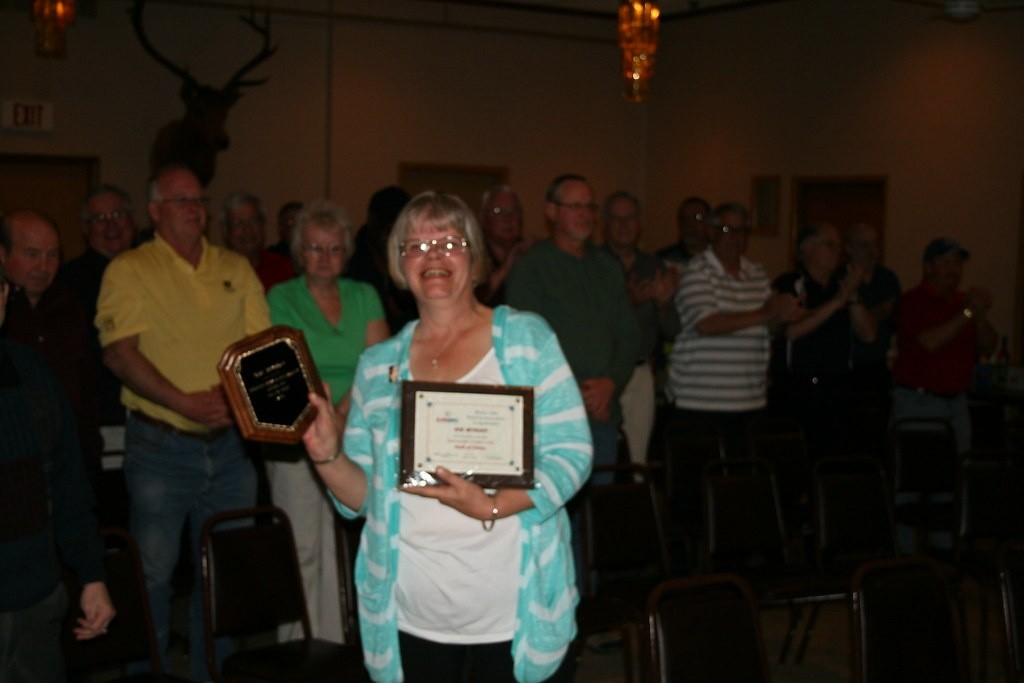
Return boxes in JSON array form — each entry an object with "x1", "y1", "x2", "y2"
[{"x1": 137, "y1": 412, "x2": 228, "y2": 441}]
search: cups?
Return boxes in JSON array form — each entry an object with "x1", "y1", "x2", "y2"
[{"x1": 1007, "y1": 366, "x2": 1024, "y2": 390}]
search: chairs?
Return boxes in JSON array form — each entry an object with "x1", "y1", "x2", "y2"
[{"x1": 59, "y1": 363, "x2": 1024, "y2": 682}]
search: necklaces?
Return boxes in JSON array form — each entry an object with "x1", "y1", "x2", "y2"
[{"x1": 430, "y1": 340, "x2": 450, "y2": 370}]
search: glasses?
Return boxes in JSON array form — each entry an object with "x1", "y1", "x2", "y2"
[
  {"x1": 86, "y1": 209, "x2": 130, "y2": 224},
  {"x1": 159, "y1": 195, "x2": 211, "y2": 209},
  {"x1": 713, "y1": 224, "x2": 751, "y2": 237},
  {"x1": 557, "y1": 202, "x2": 599, "y2": 215},
  {"x1": 301, "y1": 243, "x2": 345, "y2": 257},
  {"x1": 401, "y1": 236, "x2": 471, "y2": 257}
]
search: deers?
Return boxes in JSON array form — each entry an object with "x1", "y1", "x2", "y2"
[{"x1": 127, "y1": 0, "x2": 282, "y2": 198}]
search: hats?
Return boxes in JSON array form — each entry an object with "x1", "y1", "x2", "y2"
[{"x1": 923, "y1": 238, "x2": 970, "y2": 268}]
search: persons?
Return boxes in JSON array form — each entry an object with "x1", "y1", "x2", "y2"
[
  {"x1": 476, "y1": 175, "x2": 996, "y2": 655},
  {"x1": 0, "y1": 167, "x2": 421, "y2": 683},
  {"x1": 300, "y1": 193, "x2": 594, "y2": 683}
]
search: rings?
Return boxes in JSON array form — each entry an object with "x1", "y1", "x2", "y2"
[{"x1": 103, "y1": 629, "x2": 107, "y2": 634}]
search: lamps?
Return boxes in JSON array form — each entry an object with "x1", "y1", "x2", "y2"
[
  {"x1": 942, "y1": 0, "x2": 983, "y2": 22},
  {"x1": 617, "y1": 0, "x2": 662, "y2": 105}
]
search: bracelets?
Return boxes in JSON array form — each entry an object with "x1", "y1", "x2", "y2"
[
  {"x1": 482, "y1": 494, "x2": 497, "y2": 531},
  {"x1": 961, "y1": 307, "x2": 976, "y2": 320},
  {"x1": 311, "y1": 446, "x2": 341, "y2": 464}
]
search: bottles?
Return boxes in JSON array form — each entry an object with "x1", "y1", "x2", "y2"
[{"x1": 993, "y1": 335, "x2": 1010, "y2": 388}]
